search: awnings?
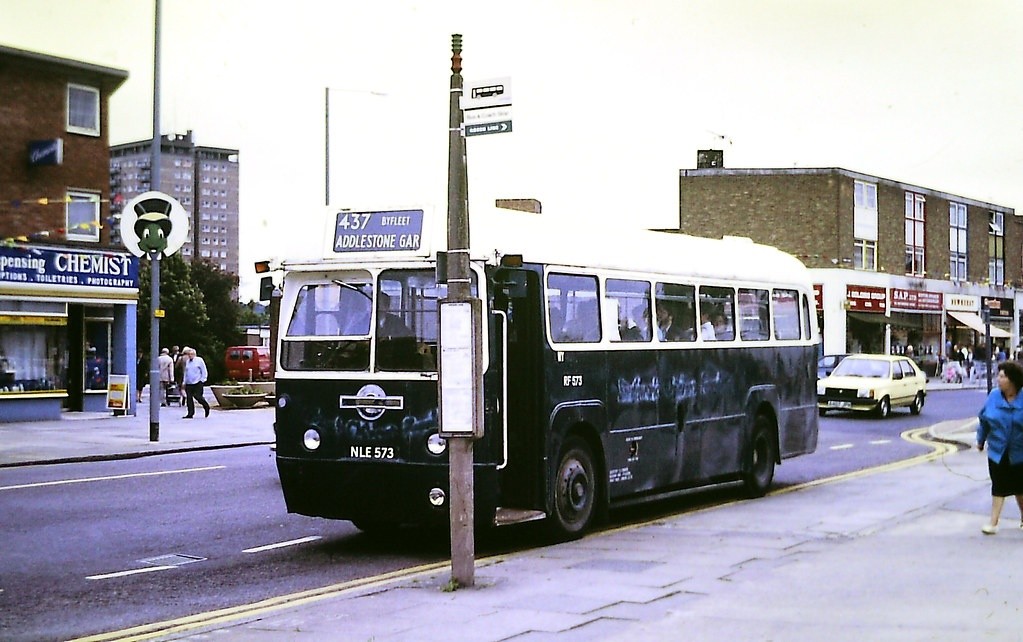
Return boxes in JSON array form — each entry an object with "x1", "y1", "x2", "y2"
[
  {"x1": 948, "y1": 311, "x2": 1010, "y2": 337},
  {"x1": 847, "y1": 312, "x2": 922, "y2": 327}
]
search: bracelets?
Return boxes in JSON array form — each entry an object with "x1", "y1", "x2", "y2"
[{"x1": 388, "y1": 335, "x2": 392, "y2": 341}]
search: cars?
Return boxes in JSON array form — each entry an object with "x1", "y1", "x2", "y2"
[{"x1": 817, "y1": 352, "x2": 929, "y2": 419}]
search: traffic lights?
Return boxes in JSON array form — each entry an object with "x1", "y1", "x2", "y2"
[{"x1": 255, "y1": 261, "x2": 269, "y2": 274}]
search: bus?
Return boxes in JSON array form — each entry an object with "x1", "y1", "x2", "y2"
[
  {"x1": 273, "y1": 234, "x2": 819, "y2": 540},
  {"x1": 225, "y1": 345, "x2": 272, "y2": 379}
]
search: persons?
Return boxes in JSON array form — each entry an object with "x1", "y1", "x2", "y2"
[
  {"x1": 980, "y1": 343, "x2": 1006, "y2": 379},
  {"x1": 905, "y1": 344, "x2": 914, "y2": 360},
  {"x1": 182, "y1": 349, "x2": 210, "y2": 418},
  {"x1": 159, "y1": 346, "x2": 190, "y2": 407},
  {"x1": 946, "y1": 339, "x2": 974, "y2": 383},
  {"x1": 976, "y1": 361, "x2": 1023, "y2": 534},
  {"x1": 549, "y1": 299, "x2": 734, "y2": 341},
  {"x1": 136, "y1": 348, "x2": 149, "y2": 403},
  {"x1": 340, "y1": 291, "x2": 410, "y2": 370}
]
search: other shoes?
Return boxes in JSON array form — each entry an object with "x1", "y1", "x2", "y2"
[
  {"x1": 983, "y1": 525, "x2": 997, "y2": 534},
  {"x1": 139, "y1": 397, "x2": 142, "y2": 403},
  {"x1": 205, "y1": 410, "x2": 209, "y2": 417},
  {"x1": 182, "y1": 397, "x2": 186, "y2": 406},
  {"x1": 183, "y1": 415, "x2": 194, "y2": 418}
]
